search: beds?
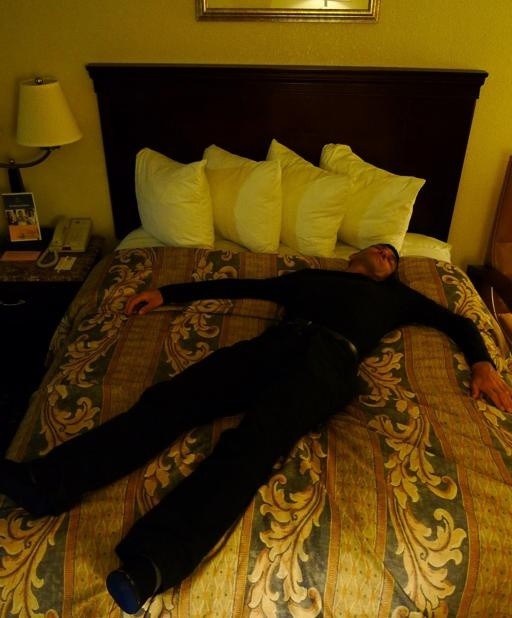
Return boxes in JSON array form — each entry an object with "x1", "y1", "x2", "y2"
[{"x1": 0, "y1": 63, "x2": 512, "y2": 616}]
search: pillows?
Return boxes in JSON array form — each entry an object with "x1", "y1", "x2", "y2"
[
  {"x1": 266, "y1": 139, "x2": 354, "y2": 258},
  {"x1": 319, "y1": 145, "x2": 426, "y2": 256},
  {"x1": 134, "y1": 148, "x2": 215, "y2": 249},
  {"x1": 203, "y1": 145, "x2": 283, "y2": 255}
]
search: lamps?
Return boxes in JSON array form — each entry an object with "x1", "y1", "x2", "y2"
[{"x1": 1, "y1": 76, "x2": 83, "y2": 252}]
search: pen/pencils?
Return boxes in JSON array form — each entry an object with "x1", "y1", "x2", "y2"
[{"x1": 57, "y1": 256, "x2": 69, "y2": 274}]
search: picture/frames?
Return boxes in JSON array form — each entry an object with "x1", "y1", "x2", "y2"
[{"x1": 193, "y1": 1, "x2": 382, "y2": 25}]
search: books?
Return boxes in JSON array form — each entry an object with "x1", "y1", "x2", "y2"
[
  {"x1": 1, "y1": 243, "x2": 512, "y2": 614},
  {"x1": 3, "y1": 193, "x2": 42, "y2": 241}
]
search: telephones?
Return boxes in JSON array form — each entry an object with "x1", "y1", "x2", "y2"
[{"x1": 36, "y1": 215, "x2": 92, "y2": 267}]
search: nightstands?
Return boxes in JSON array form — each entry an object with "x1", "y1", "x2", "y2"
[{"x1": 0, "y1": 235, "x2": 108, "y2": 373}]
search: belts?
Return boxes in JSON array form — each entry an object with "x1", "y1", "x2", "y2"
[{"x1": 285, "y1": 319, "x2": 359, "y2": 359}]
